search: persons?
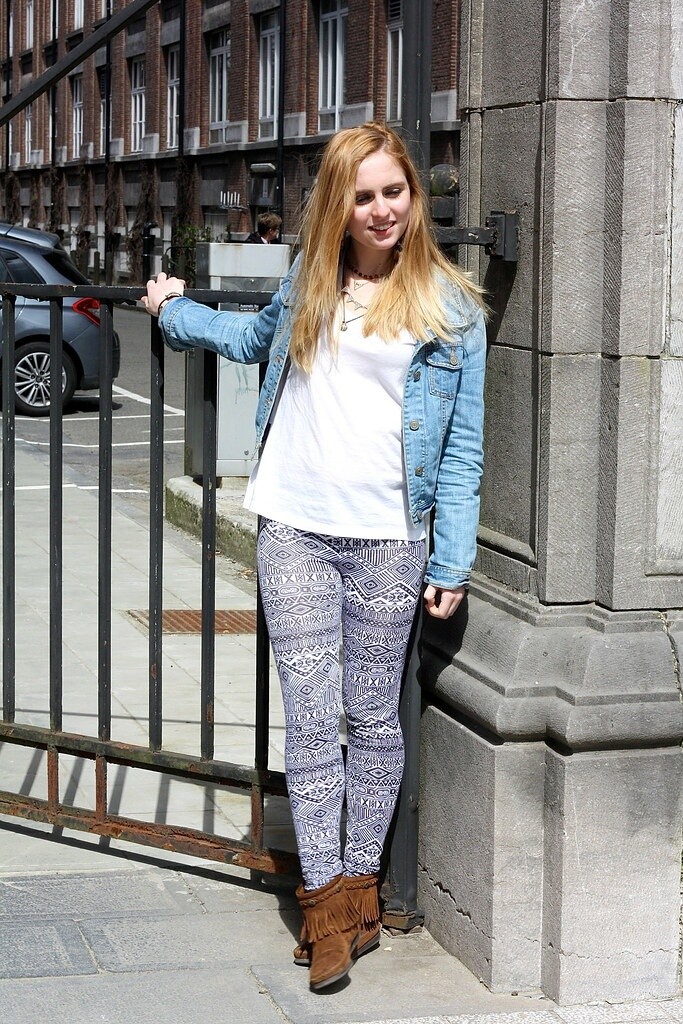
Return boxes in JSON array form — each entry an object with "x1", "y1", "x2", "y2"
[
  {"x1": 245, "y1": 212, "x2": 282, "y2": 244},
  {"x1": 141, "y1": 122, "x2": 487, "y2": 994}
]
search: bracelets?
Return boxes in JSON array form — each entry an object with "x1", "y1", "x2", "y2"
[{"x1": 157, "y1": 292, "x2": 182, "y2": 317}]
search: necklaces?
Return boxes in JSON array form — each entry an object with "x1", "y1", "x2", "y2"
[{"x1": 341, "y1": 260, "x2": 390, "y2": 331}]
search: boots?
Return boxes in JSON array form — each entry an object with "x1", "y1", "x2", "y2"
[{"x1": 293, "y1": 873, "x2": 382, "y2": 991}]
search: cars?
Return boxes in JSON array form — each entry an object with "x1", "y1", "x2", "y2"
[
  {"x1": 0, "y1": 219, "x2": 74, "y2": 264},
  {"x1": 0, "y1": 237, "x2": 122, "y2": 417}
]
